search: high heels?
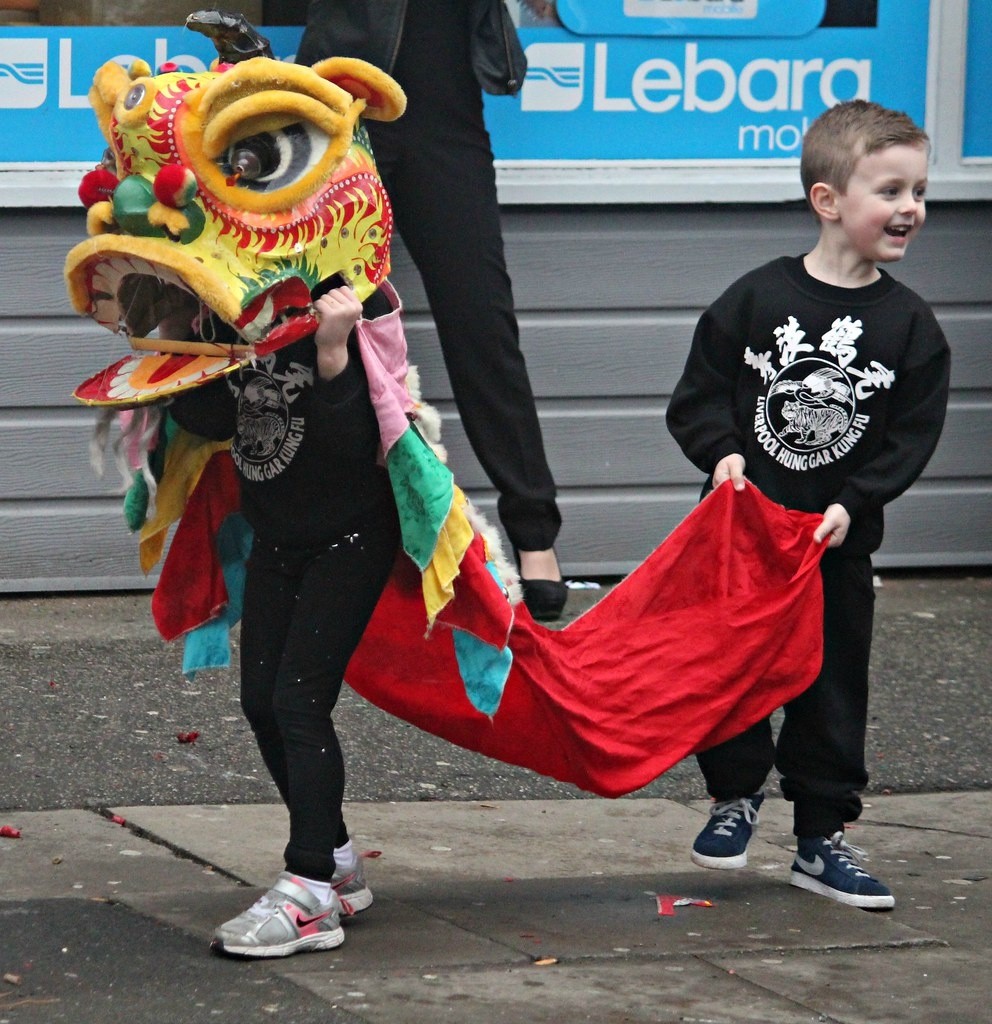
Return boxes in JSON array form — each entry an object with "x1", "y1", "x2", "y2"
[{"x1": 512, "y1": 546, "x2": 570, "y2": 620}]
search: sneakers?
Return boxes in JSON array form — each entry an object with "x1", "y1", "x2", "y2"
[
  {"x1": 690, "y1": 790, "x2": 766, "y2": 870},
  {"x1": 332, "y1": 855, "x2": 374, "y2": 915},
  {"x1": 209, "y1": 871, "x2": 347, "y2": 958},
  {"x1": 788, "y1": 831, "x2": 896, "y2": 909}
]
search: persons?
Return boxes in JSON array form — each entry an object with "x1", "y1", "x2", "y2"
[
  {"x1": 286, "y1": 1, "x2": 572, "y2": 626},
  {"x1": 661, "y1": 99, "x2": 951, "y2": 908},
  {"x1": 147, "y1": 182, "x2": 429, "y2": 962}
]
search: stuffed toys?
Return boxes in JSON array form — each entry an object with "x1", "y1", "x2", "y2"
[{"x1": 57, "y1": 50, "x2": 408, "y2": 417}]
